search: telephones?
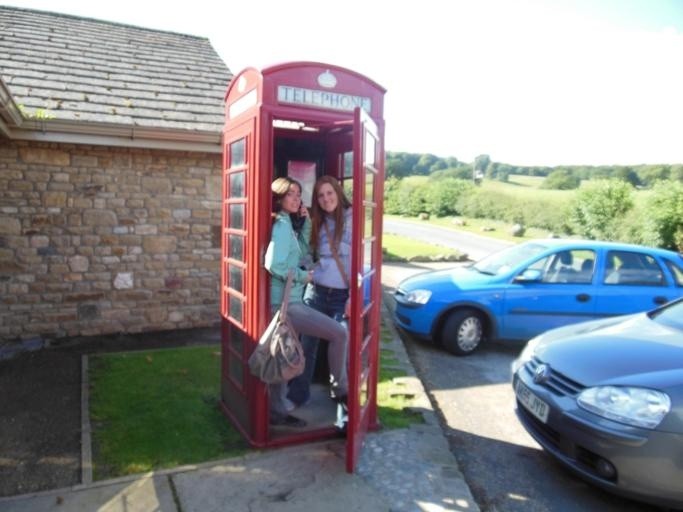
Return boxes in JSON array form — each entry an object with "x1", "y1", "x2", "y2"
[{"x1": 293, "y1": 200, "x2": 306, "y2": 230}]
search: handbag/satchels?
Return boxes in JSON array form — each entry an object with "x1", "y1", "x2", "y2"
[{"x1": 248, "y1": 308, "x2": 307, "y2": 385}]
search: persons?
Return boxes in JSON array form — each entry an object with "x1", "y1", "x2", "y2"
[
  {"x1": 262, "y1": 175, "x2": 350, "y2": 427},
  {"x1": 283, "y1": 174, "x2": 352, "y2": 413}
]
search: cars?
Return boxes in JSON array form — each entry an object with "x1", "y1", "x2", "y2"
[
  {"x1": 509, "y1": 295, "x2": 682, "y2": 511},
  {"x1": 390, "y1": 238, "x2": 682, "y2": 356}
]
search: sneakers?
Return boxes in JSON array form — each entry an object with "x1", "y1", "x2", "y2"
[
  {"x1": 269, "y1": 410, "x2": 305, "y2": 431},
  {"x1": 283, "y1": 396, "x2": 310, "y2": 411},
  {"x1": 328, "y1": 394, "x2": 348, "y2": 412}
]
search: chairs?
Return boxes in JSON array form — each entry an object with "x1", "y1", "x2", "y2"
[{"x1": 546, "y1": 251, "x2": 662, "y2": 284}]
